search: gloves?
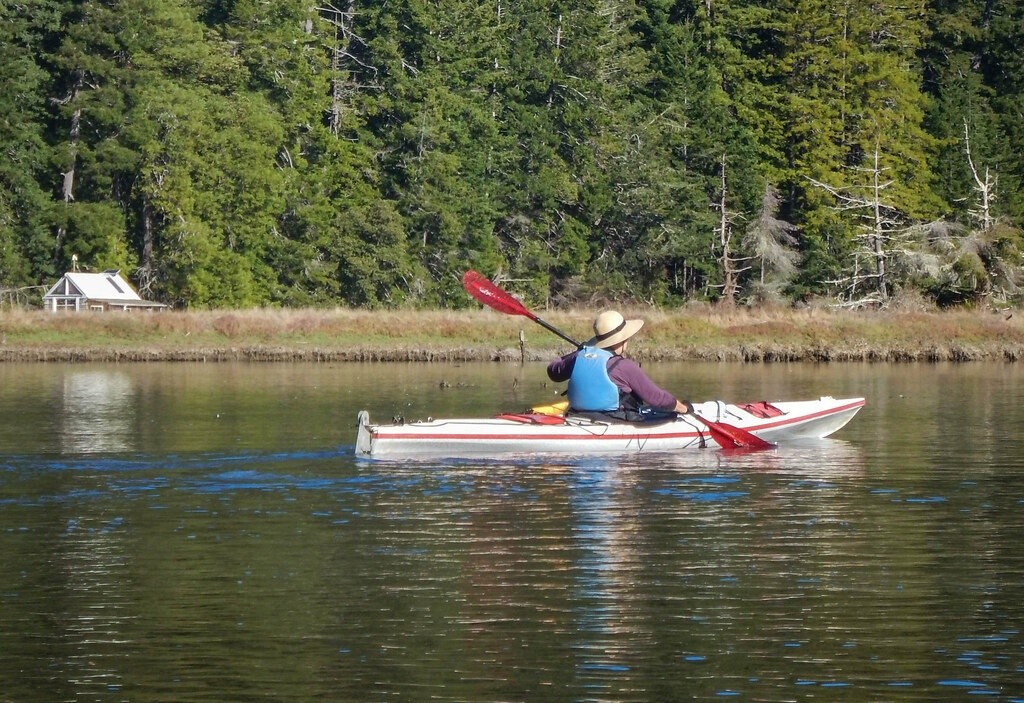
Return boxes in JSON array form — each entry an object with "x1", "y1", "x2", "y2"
[
  {"x1": 578, "y1": 342, "x2": 588, "y2": 350},
  {"x1": 677, "y1": 399, "x2": 694, "y2": 415}
]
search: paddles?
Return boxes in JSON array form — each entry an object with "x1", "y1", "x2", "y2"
[{"x1": 460, "y1": 267, "x2": 775, "y2": 452}]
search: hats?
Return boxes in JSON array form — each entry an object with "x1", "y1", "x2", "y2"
[{"x1": 584, "y1": 310, "x2": 644, "y2": 349}]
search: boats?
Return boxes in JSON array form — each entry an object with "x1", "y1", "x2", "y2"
[{"x1": 355, "y1": 396, "x2": 869, "y2": 463}]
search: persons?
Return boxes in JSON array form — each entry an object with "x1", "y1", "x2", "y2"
[{"x1": 547, "y1": 310, "x2": 693, "y2": 424}]
